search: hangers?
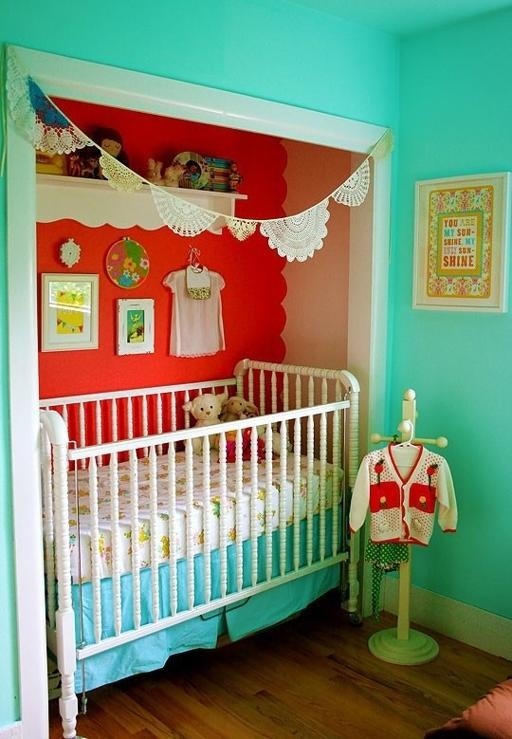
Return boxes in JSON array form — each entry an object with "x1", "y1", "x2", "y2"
[{"x1": 390, "y1": 420, "x2": 421, "y2": 456}]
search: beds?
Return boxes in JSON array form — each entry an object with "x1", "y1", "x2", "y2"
[{"x1": 39, "y1": 359, "x2": 361, "y2": 739}]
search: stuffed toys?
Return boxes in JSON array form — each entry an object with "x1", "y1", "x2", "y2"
[
  {"x1": 66, "y1": 151, "x2": 87, "y2": 176},
  {"x1": 165, "y1": 160, "x2": 184, "y2": 186},
  {"x1": 145, "y1": 159, "x2": 165, "y2": 186},
  {"x1": 256, "y1": 427, "x2": 294, "y2": 458},
  {"x1": 84, "y1": 130, "x2": 129, "y2": 180},
  {"x1": 219, "y1": 427, "x2": 267, "y2": 467},
  {"x1": 181, "y1": 392, "x2": 226, "y2": 454},
  {"x1": 219, "y1": 395, "x2": 260, "y2": 424}
]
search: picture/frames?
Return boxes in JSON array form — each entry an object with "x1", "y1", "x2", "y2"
[
  {"x1": 117, "y1": 299, "x2": 155, "y2": 356},
  {"x1": 41, "y1": 273, "x2": 100, "y2": 352},
  {"x1": 411, "y1": 171, "x2": 511, "y2": 313}
]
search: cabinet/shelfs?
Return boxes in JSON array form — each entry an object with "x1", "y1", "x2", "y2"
[{"x1": 367, "y1": 389, "x2": 448, "y2": 667}]
